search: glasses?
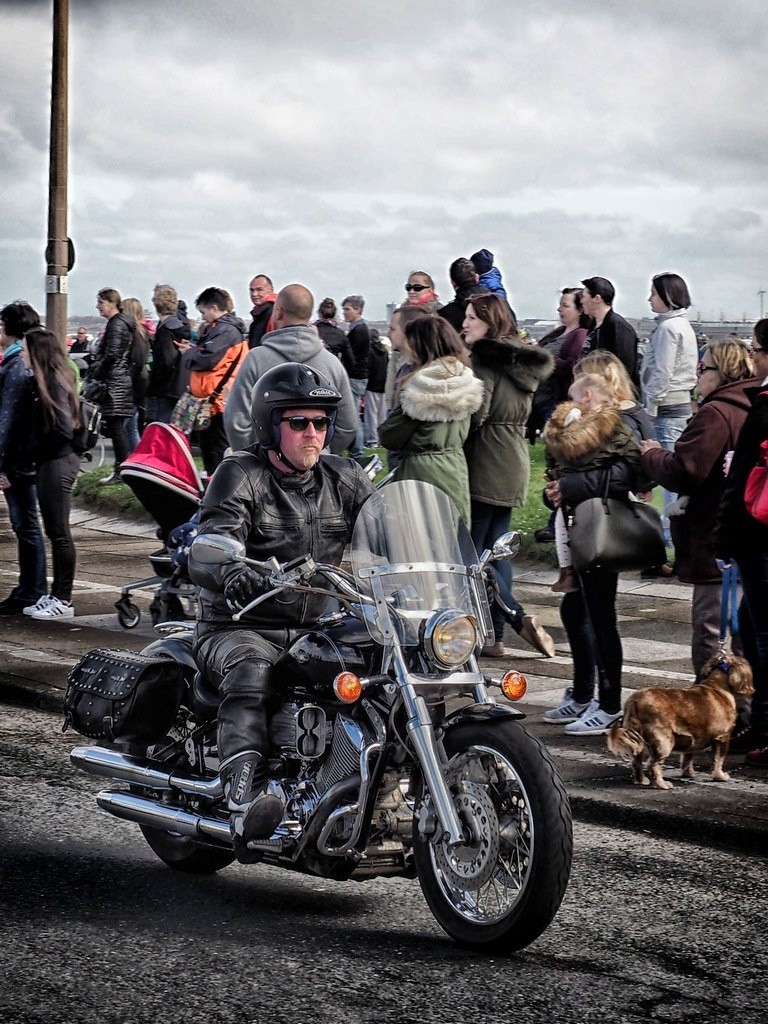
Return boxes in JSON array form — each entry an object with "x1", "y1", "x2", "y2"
[
  {"x1": 405, "y1": 284, "x2": 430, "y2": 292},
  {"x1": 701, "y1": 364, "x2": 718, "y2": 374},
  {"x1": 280, "y1": 416, "x2": 332, "y2": 431}
]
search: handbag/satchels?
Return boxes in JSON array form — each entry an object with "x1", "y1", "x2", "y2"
[
  {"x1": 169, "y1": 392, "x2": 211, "y2": 437},
  {"x1": 569, "y1": 466, "x2": 668, "y2": 571}
]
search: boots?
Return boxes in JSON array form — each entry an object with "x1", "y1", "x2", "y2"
[{"x1": 218, "y1": 751, "x2": 283, "y2": 865}]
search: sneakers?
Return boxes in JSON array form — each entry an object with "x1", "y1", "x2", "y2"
[
  {"x1": 543, "y1": 698, "x2": 593, "y2": 722},
  {"x1": 565, "y1": 699, "x2": 624, "y2": 735},
  {"x1": 23, "y1": 594, "x2": 74, "y2": 619}
]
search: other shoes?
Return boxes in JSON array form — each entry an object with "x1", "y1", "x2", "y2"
[
  {"x1": 550, "y1": 566, "x2": 579, "y2": 593},
  {"x1": 522, "y1": 614, "x2": 556, "y2": 658},
  {"x1": 483, "y1": 642, "x2": 506, "y2": 656},
  {"x1": 0, "y1": 587, "x2": 37, "y2": 617}
]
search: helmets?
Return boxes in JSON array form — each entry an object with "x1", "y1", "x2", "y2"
[{"x1": 252, "y1": 363, "x2": 343, "y2": 449}]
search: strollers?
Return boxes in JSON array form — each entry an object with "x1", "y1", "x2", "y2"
[{"x1": 114, "y1": 422, "x2": 385, "y2": 629}]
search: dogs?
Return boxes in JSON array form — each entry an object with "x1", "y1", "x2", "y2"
[{"x1": 605, "y1": 650, "x2": 755, "y2": 790}]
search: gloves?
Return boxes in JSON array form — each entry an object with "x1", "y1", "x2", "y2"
[{"x1": 224, "y1": 569, "x2": 266, "y2": 611}]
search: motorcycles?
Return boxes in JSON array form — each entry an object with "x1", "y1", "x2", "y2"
[{"x1": 70, "y1": 479, "x2": 573, "y2": 953}]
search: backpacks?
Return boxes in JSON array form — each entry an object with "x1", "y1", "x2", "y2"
[{"x1": 71, "y1": 400, "x2": 103, "y2": 461}]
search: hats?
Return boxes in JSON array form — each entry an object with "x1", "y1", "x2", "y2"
[{"x1": 470, "y1": 249, "x2": 493, "y2": 275}]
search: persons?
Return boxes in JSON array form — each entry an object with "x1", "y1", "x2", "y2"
[
  {"x1": 189, "y1": 362, "x2": 489, "y2": 864},
  {"x1": 377, "y1": 248, "x2": 768, "y2": 736},
  {"x1": 0, "y1": 305, "x2": 81, "y2": 621},
  {"x1": 68, "y1": 275, "x2": 396, "y2": 484}
]
